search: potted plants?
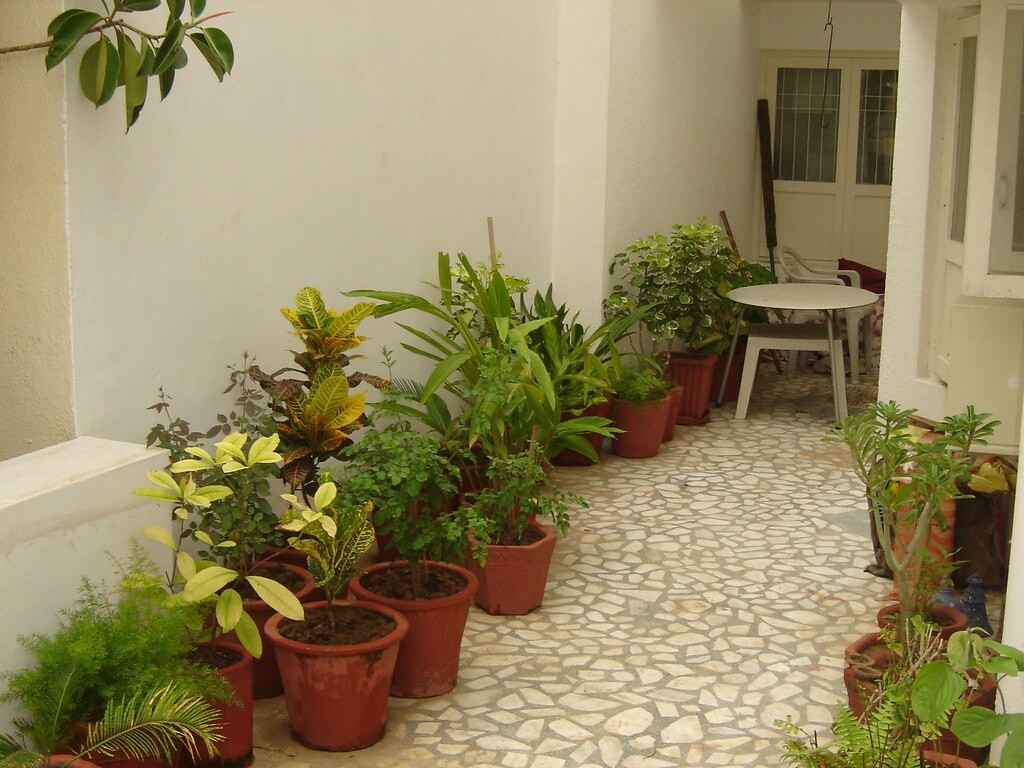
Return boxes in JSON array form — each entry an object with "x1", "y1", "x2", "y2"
[
  {"x1": 341, "y1": 346, "x2": 479, "y2": 698},
  {"x1": 610, "y1": 363, "x2": 672, "y2": 459},
  {"x1": 459, "y1": 440, "x2": 590, "y2": 616},
  {"x1": 143, "y1": 350, "x2": 317, "y2": 698},
  {"x1": 682, "y1": 246, "x2": 787, "y2": 404},
  {"x1": 262, "y1": 468, "x2": 409, "y2": 751},
  {"x1": 598, "y1": 233, "x2": 684, "y2": 445},
  {"x1": 778, "y1": 400, "x2": 1024, "y2": 768},
  {"x1": 232, "y1": 285, "x2": 378, "y2": 603},
  {"x1": 127, "y1": 445, "x2": 305, "y2": 768},
  {"x1": 611, "y1": 214, "x2": 751, "y2": 419},
  {"x1": 0, "y1": 534, "x2": 245, "y2": 768},
  {"x1": 511, "y1": 281, "x2": 668, "y2": 470},
  {"x1": 0, "y1": 676, "x2": 231, "y2": 768},
  {"x1": 352, "y1": 416, "x2": 443, "y2": 559},
  {"x1": 445, "y1": 251, "x2": 553, "y2": 486}
]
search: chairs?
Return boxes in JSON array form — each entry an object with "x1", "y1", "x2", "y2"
[{"x1": 777, "y1": 244, "x2": 873, "y2": 385}]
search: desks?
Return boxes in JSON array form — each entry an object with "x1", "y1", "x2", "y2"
[{"x1": 726, "y1": 283, "x2": 880, "y2": 432}]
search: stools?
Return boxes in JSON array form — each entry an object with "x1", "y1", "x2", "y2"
[{"x1": 733, "y1": 322, "x2": 849, "y2": 428}]
